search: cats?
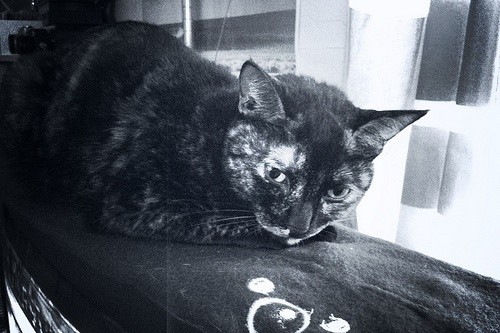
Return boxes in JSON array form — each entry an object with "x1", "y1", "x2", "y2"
[{"x1": 0, "y1": 19, "x2": 429, "y2": 251}]
